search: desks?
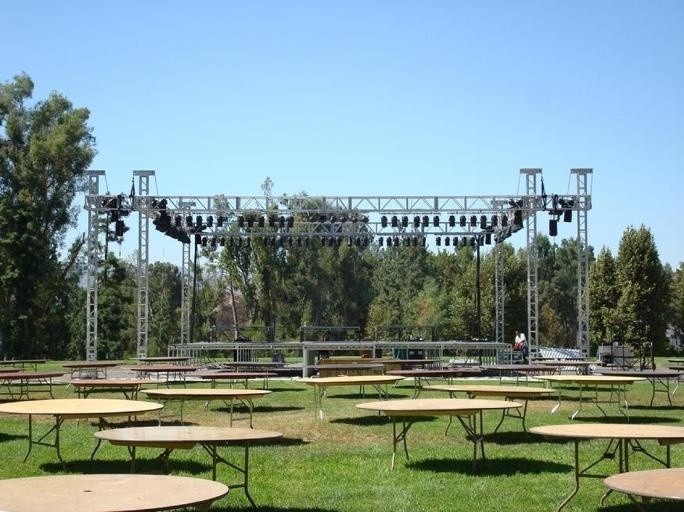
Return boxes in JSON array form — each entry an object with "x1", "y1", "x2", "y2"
[{"x1": 0, "y1": 356, "x2": 684, "y2": 512}]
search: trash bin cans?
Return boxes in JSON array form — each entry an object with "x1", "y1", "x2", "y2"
[{"x1": 233, "y1": 335, "x2": 251, "y2": 362}]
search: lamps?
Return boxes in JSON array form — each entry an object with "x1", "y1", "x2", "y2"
[
  {"x1": 114, "y1": 220, "x2": 125, "y2": 237},
  {"x1": 170, "y1": 210, "x2": 524, "y2": 230},
  {"x1": 548, "y1": 199, "x2": 574, "y2": 237},
  {"x1": 192, "y1": 234, "x2": 494, "y2": 250}
]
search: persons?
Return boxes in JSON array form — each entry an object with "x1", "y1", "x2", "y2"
[{"x1": 512, "y1": 326, "x2": 527, "y2": 356}]
